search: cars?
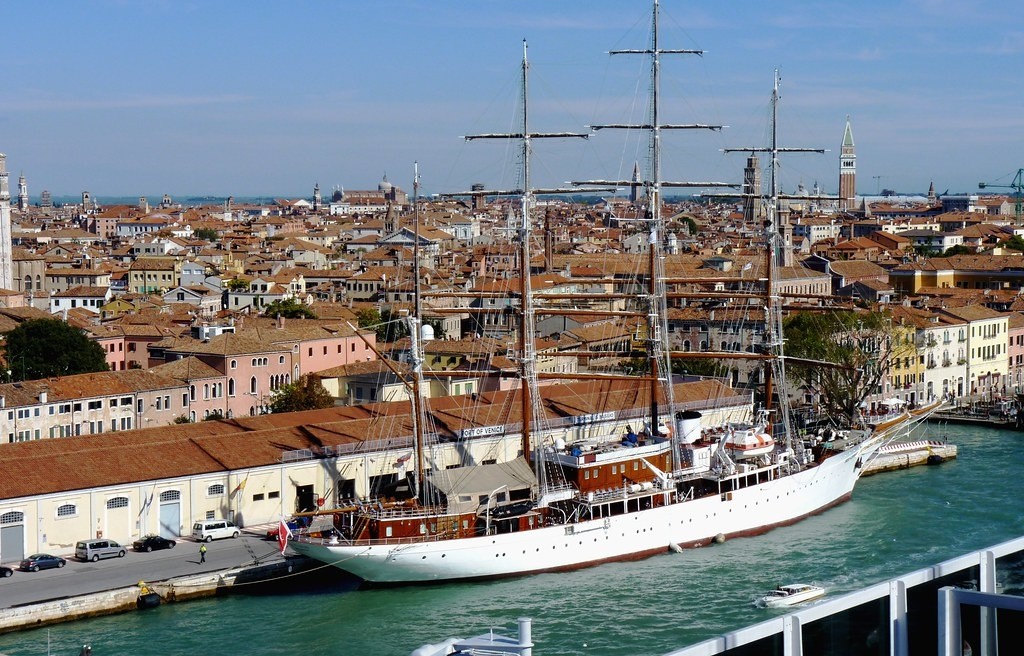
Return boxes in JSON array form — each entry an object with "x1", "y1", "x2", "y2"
[
  {"x1": 0, "y1": 566, "x2": 14, "y2": 578},
  {"x1": 133, "y1": 535, "x2": 176, "y2": 552},
  {"x1": 19, "y1": 553, "x2": 67, "y2": 572}
]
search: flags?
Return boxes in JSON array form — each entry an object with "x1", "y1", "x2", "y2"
[
  {"x1": 397, "y1": 452, "x2": 412, "y2": 462},
  {"x1": 279, "y1": 517, "x2": 290, "y2": 556},
  {"x1": 229, "y1": 480, "x2": 247, "y2": 500}
]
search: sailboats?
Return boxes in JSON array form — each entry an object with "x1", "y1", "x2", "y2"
[{"x1": 262, "y1": 0, "x2": 952, "y2": 585}]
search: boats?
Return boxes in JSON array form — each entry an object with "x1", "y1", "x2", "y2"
[
  {"x1": 408, "y1": 616, "x2": 534, "y2": 656},
  {"x1": 751, "y1": 580, "x2": 826, "y2": 607},
  {"x1": 477, "y1": 500, "x2": 534, "y2": 519}
]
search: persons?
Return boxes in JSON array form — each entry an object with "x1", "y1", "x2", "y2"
[{"x1": 199, "y1": 544, "x2": 206, "y2": 563}]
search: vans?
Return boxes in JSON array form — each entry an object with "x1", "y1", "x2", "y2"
[
  {"x1": 74, "y1": 538, "x2": 128, "y2": 562},
  {"x1": 192, "y1": 519, "x2": 241, "y2": 543}
]
